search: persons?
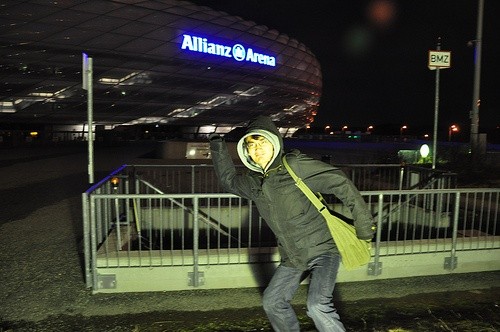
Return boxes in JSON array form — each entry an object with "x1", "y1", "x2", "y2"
[{"x1": 209, "y1": 115, "x2": 377, "y2": 332}]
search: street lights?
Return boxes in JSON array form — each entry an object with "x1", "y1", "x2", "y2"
[{"x1": 305, "y1": 126, "x2": 458, "y2": 136}]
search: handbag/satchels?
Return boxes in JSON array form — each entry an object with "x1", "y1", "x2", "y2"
[{"x1": 326, "y1": 215, "x2": 372, "y2": 271}]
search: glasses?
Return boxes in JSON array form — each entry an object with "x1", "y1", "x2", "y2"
[{"x1": 244, "y1": 139, "x2": 268, "y2": 148}]
